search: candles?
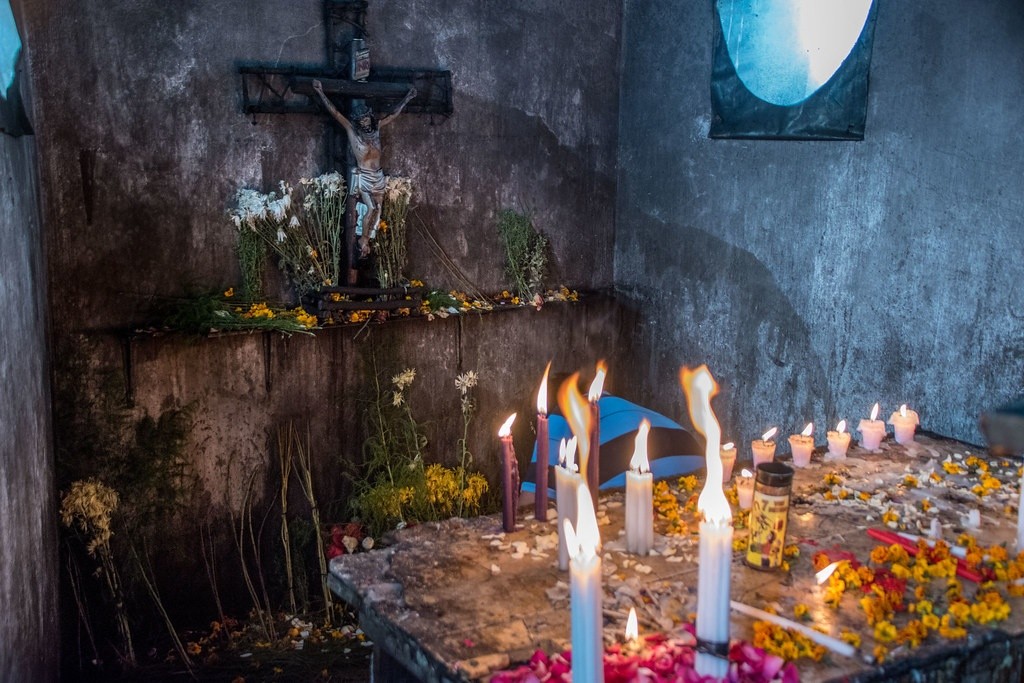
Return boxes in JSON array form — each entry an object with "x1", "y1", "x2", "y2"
[
  {"x1": 826, "y1": 420, "x2": 852, "y2": 461},
  {"x1": 888, "y1": 404, "x2": 919, "y2": 444},
  {"x1": 498, "y1": 354, "x2": 737, "y2": 683},
  {"x1": 736, "y1": 470, "x2": 759, "y2": 509},
  {"x1": 858, "y1": 402, "x2": 886, "y2": 449},
  {"x1": 752, "y1": 427, "x2": 778, "y2": 472},
  {"x1": 861, "y1": 526, "x2": 993, "y2": 584},
  {"x1": 326, "y1": 434, "x2": 1024, "y2": 683},
  {"x1": 726, "y1": 601, "x2": 858, "y2": 657},
  {"x1": 788, "y1": 424, "x2": 817, "y2": 467}
]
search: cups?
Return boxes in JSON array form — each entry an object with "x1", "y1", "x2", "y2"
[{"x1": 744, "y1": 461, "x2": 793, "y2": 572}]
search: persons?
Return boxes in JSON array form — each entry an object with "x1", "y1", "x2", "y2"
[
  {"x1": 312, "y1": 79, "x2": 418, "y2": 256},
  {"x1": 516, "y1": 371, "x2": 705, "y2": 510}
]
